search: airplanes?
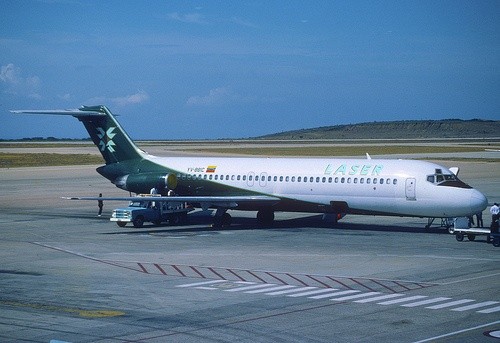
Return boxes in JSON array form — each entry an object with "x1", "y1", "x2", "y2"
[{"x1": 8, "y1": 103, "x2": 490, "y2": 235}]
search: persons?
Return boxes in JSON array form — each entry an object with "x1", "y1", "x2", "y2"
[
  {"x1": 97, "y1": 193, "x2": 103, "y2": 216},
  {"x1": 468, "y1": 203, "x2": 500, "y2": 227},
  {"x1": 150, "y1": 184, "x2": 176, "y2": 197}
]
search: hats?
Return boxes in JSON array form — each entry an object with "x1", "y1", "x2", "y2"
[{"x1": 99, "y1": 193, "x2": 102, "y2": 195}]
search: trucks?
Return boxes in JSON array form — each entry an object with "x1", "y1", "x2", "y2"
[{"x1": 110, "y1": 194, "x2": 195, "y2": 229}]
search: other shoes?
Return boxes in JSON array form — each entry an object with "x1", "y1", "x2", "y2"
[{"x1": 98, "y1": 215, "x2": 101, "y2": 217}]
